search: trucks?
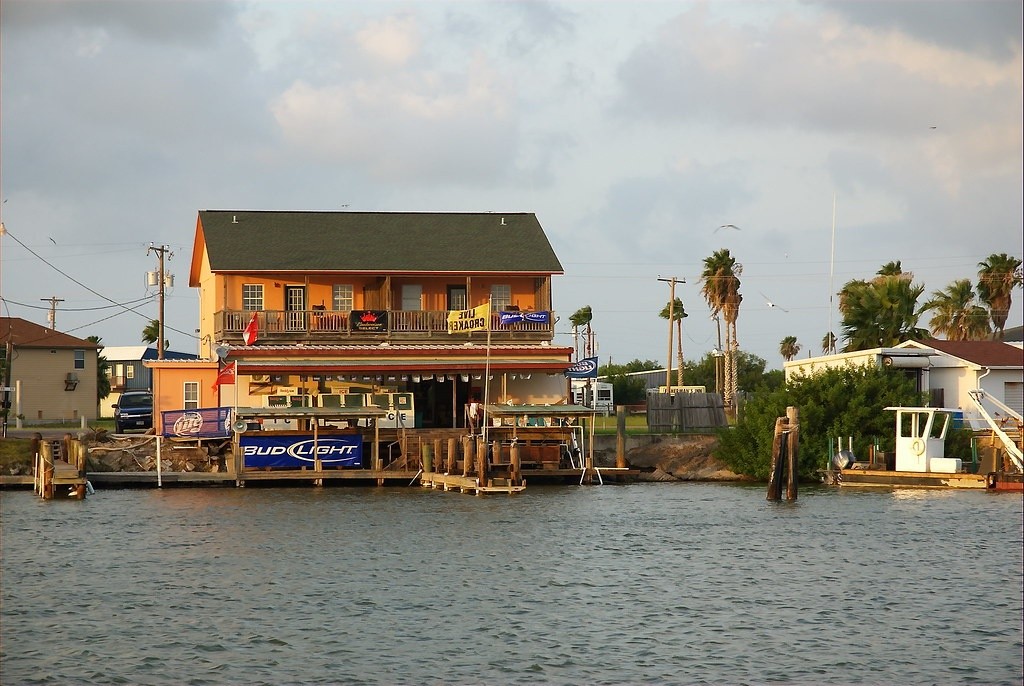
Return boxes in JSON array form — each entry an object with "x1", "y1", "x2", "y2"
[{"x1": 571, "y1": 378, "x2": 613, "y2": 414}]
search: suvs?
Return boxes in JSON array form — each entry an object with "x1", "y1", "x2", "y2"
[{"x1": 112, "y1": 391, "x2": 153, "y2": 434}]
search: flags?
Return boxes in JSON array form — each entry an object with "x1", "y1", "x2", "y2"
[
  {"x1": 242, "y1": 311, "x2": 258, "y2": 345},
  {"x1": 212, "y1": 361, "x2": 235, "y2": 395},
  {"x1": 447, "y1": 302, "x2": 488, "y2": 334},
  {"x1": 563, "y1": 357, "x2": 598, "y2": 379}
]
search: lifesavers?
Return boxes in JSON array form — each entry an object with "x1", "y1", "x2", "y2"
[{"x1": 911, "y1": 439, "x2": 925, "y2": 456}]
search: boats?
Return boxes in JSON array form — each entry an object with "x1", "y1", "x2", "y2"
[
  {"x1": 981, "y1": 470, "x2": 1023, "y2": 493},
  {"x1": 841, "y1": 406, "x2": 989, "y2": 490}
]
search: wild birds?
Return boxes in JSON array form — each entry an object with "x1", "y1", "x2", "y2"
[
  {"x1": 760, "y1": 293, "x2": 789, "y2": 313},
  {"x1": 714, "y1": 224, "x2": 741, "y2": 234}
]
